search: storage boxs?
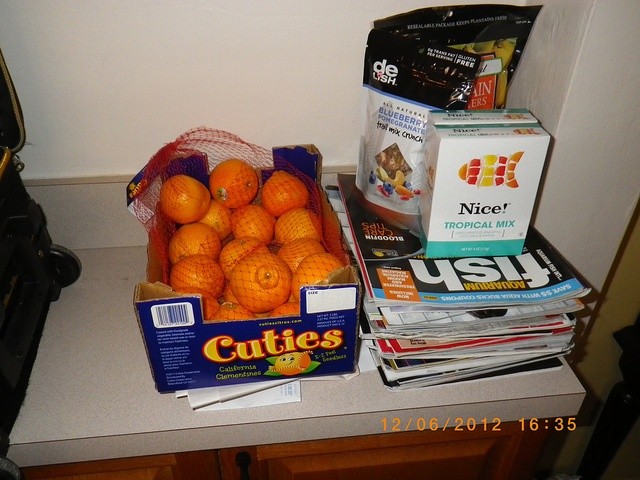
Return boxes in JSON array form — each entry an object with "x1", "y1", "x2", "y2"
[{"x1": 133, "y1": 143, "x2": 364, "y2": 396}]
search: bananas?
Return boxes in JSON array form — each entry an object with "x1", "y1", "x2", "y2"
[
  {"x1": 376, "y1": 166, "x2": 405, "y2": 188},
  {"x1": 453, "y1": 41, "x2": 518, "y2": 107}
]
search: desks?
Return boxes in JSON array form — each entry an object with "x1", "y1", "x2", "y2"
[{"x1": 7, "y1": 164, "x2": 586, "y2": 468}]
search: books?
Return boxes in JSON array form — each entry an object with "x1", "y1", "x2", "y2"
[{"x1": 326, "y1": 173, "x2": 592, "y2": 391}]
